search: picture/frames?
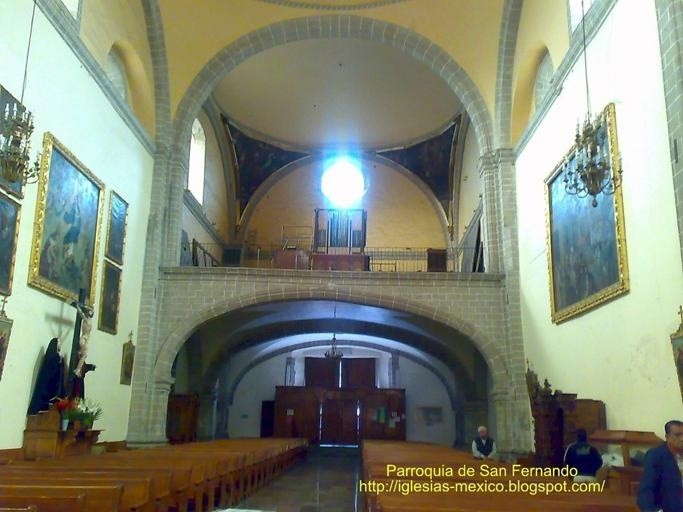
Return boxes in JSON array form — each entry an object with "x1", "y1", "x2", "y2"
[{"x1": 542, "y1": 101, "x2": 631, "y2": 324}]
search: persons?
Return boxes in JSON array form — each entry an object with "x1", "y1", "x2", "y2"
[
  {"x1": 72, "y1": 303, "x2": 94, "y2": 377},
  {"x1": 38, "y1": 337, "x2": 65, "y2": 413},
  {"x1": 562, "y1": 427, "x2": 603, "y2": 483},
  {"x1": 470, "y1": 426, "x2": 496, "y2": 461},
  {"x1": 634, "y1": 420, "x2": 683, "y2": 512}
]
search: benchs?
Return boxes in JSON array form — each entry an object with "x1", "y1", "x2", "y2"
[{"x1": 359, "y1": 438, "x2": 645, "y2": 512}]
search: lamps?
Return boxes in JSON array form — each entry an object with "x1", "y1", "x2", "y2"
[
  {"x1": 0, "y1": 0, "x2": 42, "y2": 196},
  {"x1": 559, "y1": 1, "x2": 623, "y2": 208}
]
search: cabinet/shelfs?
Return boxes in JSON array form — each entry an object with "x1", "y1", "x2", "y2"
[{"x1": 307, "y1": 255, "x2": 370, "y2": 270}]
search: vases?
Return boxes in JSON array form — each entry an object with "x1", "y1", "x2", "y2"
[{"x1": 61, "y1": 418, "x2": 81, "y2": 431}]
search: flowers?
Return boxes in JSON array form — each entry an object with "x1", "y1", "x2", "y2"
[{"x1": 55, "y1": 396, "x2": 104, "y2": 427}]
